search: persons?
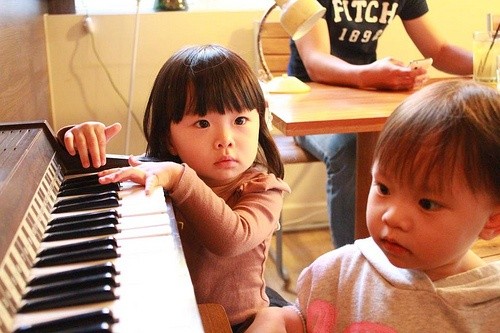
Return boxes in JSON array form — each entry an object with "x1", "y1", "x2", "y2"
[
  {"x1": 287, "y1": 0, "x2": 474, "y2": 249},
  {"x1": 246, "y1": 79, "x2": 500, "y2": 333},
  {"x1": 57, "y1": 44, "x2": 294, "y2": 333}
]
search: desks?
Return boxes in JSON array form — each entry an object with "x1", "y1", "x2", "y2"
[{"x1": 262, "y1": 75, "x2": 500, "y2": 243}]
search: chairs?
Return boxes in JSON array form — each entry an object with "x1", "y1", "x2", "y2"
[{"x1": 255, "y1": 21, "x2": 324, "y2": 281}]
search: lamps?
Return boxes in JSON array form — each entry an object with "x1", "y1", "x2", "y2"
[{"x1": 256, "y1": 0, "x2": 326, "y2": 94}]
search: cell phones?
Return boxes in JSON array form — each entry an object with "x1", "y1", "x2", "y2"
[{"x1": 406, "y1": 58, "x2": 434, "y2": 79}]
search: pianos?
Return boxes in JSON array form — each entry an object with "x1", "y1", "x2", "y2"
[{"x1": 0, "y1": 121, "x2": 207, "y2": 332}]
